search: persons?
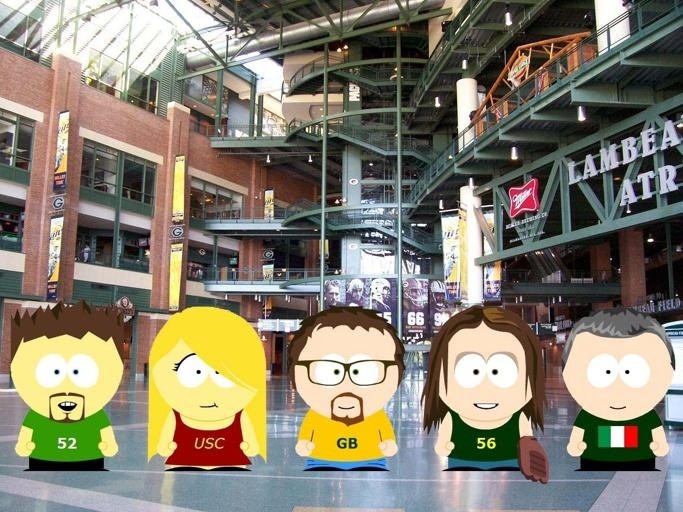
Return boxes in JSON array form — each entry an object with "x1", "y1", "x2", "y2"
[
  {"x1": 80, "y1": 243, "x2": 90, "y2": 262},
  {"x1": 347, "y1": 279, "x2": 369, "y2": 307},
  {"x1": 370, "y1": 278, "x2": 391, "y2": 312},
  {"x1": 401, "y1": 278, "x2": 425, "y2": 311},
  {"x1": 323, "y1": 280, "x2": 344, "y2": 310},
  {"x1": 428, "y1": 279, "x2": 450, "y2": 308}
]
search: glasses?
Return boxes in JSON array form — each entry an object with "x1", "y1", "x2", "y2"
[{"x1": 295, "y1": 359, "x2": 398, "y2": 387}]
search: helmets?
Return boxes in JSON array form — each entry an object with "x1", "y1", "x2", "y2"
[
  {"x1": 348, "y1": 279, "x2": 363, "y2": 301},
  {"x1": 402, "y1": 279, "x2": 424, "y2": 307},
  {"x1": 371, "y1": 278, "x2": 391, "y2": 302},
  {"x1": 431, "y1": 280, "x2": 447, "y2": 308}
]
